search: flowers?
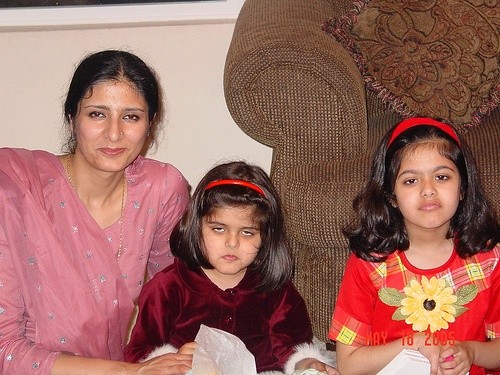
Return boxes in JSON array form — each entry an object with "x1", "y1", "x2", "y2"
[{"x1": 378, "y1": 275, "x2": 478, "y2": 333}]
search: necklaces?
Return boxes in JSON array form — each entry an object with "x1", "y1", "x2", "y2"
[{"x1": 66, "y1": 154, "x2": 126, "y2": 263}]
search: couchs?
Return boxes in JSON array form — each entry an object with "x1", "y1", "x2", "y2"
[{"x1": 225, "y1": 0, "x2": 499, "y2": 345}]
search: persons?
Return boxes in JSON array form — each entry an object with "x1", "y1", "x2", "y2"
[
  {"x1": 326, "y1": 115, "x2": 500, "y2": 375},
  {"x1": 123, "y1": 162, "x2": 342, "y2": 374},
  {"x1": 0, "y1": 48, "x2": 202, "y2": 375}
]
